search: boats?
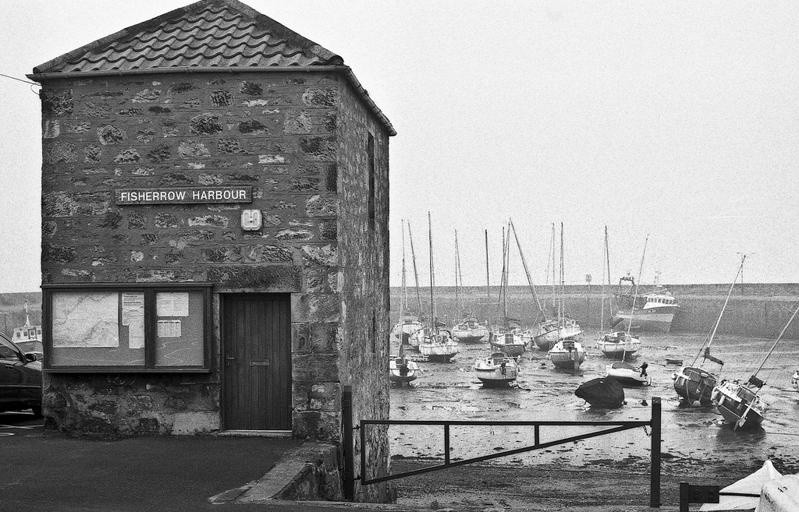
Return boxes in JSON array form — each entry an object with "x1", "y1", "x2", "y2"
[
  {"x1": 12, "y1": 298, "x2": 44, "y2": 354},
  {"x1": 697, "y1": 454, "x2": 799, "y2": 512}
]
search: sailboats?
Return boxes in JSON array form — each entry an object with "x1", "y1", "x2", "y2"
[
  {"x1": 708, "y1": 307, "x2": 799, "y2": 431},
  {"x1": 669, "y1": 254, "x2": 749, "y2": 409},
  {"x1": 388, "y1": 211, "x2": 675, "y2": 409}
]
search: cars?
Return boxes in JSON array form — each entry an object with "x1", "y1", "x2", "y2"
[{"x1": 0, "y1": 333, "x2": 44, "y2": 417}]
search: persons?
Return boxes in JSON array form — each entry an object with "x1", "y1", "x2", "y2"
[
  {"x1": 638, "y1": 361, "x2": 648, "y2": 378},
  {"x1": 500, "y1": 360, "x2": 506, "y2": 375}
]
